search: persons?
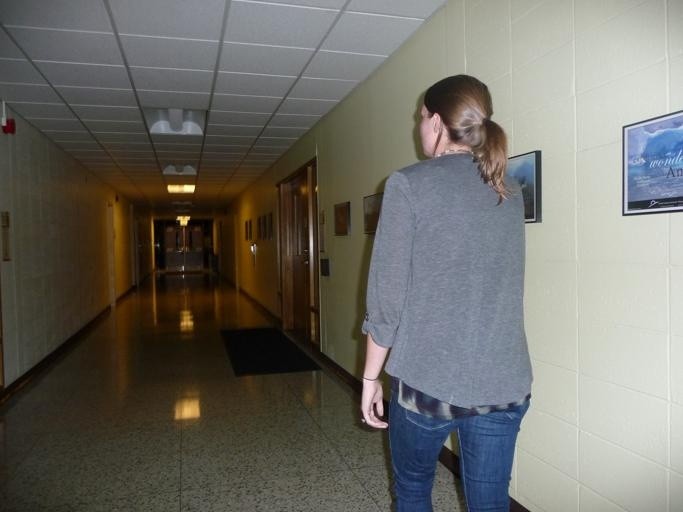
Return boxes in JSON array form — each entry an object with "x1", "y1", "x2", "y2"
[{"x1": 362, "y1": 74, "x2": 532, "y2": 511}]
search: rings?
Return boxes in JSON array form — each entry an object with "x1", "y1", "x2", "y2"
[{"x1": 361, "y1": 418, "x2": 365, "y2": 423}]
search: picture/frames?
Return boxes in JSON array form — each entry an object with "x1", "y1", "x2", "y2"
[
  {"x1": 363, "y1": 192, "x2": 383, "y2": 234},
  {"x1": 506, "y1": 151, "x2": 542, "y2": 223},
  {"x1": 334, "y1": 201, "x2": 351, "y2": 237},
  {"x1": 622, "y1": 110, "x2": 683, "y2": 217}
]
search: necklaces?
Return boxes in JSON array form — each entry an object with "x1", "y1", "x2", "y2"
[{"x1": 437, "y1": 148, "x2": 471, "y2": 155}]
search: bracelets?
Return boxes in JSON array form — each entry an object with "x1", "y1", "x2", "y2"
[{"x1": 363, "y1": 376, "x2": 379, "y2": 382}]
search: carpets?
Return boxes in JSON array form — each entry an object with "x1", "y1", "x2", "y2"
[{"x1": 220, "y1": 326, "x2": 323, "y2": 378}]
